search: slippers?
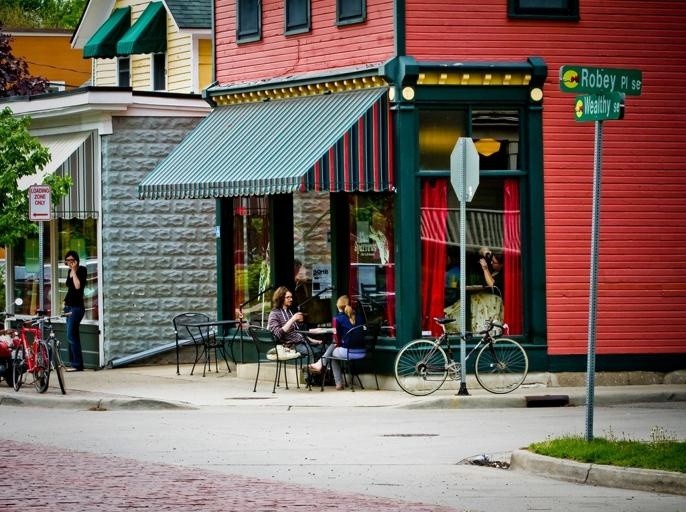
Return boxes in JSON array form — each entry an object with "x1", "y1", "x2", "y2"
[{"x1": 303, "y1": 363, "x2": 321, "y2": 374}]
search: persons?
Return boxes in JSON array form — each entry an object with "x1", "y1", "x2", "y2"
[
  {"x1": 266, "y1": 286, "x2": 329, "y2": 386},
  {"x1": 63, "y1": 251, "x2": 87, "y2": 373},
  {"x1": 443, "y1": 247, "x2": 505, "y2": 335},
  {"x1": 442, "y1": 245, "x2": 481, "y2": 306},
  {"x1": 302, "y1": 294, "x2": 369, "y2": 390}
]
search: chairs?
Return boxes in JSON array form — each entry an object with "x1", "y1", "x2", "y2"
[
  {"x1": 173, "y1": 311, "x2": 211, "y2": 375},
  {"x1": 248, "y1": 323, "x2": 379, "y2": 394}
]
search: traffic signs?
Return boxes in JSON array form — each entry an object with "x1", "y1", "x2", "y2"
[
  {"x1": 555, "y1": 60, "x2": 643, "y2": 124},
  {"x1": 28, "y1": 185, "x2": 52, "y2": 222}
]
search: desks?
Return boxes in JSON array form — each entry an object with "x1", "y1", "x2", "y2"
[{"x1": 181, "y1": 319, "x2": 247, "y2": 376}]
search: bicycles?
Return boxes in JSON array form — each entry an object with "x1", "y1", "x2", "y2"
[
  {"x1": 14, "y1": 311, "x2": 72, "y2": 395},
  {"x1": 393, "y1": 316, "x2": 530, "y2": 396}
]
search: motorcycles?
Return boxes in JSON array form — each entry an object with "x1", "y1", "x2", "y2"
[{"x1": 0, "y1": 309, "x2": 22, "y2": 388}]
